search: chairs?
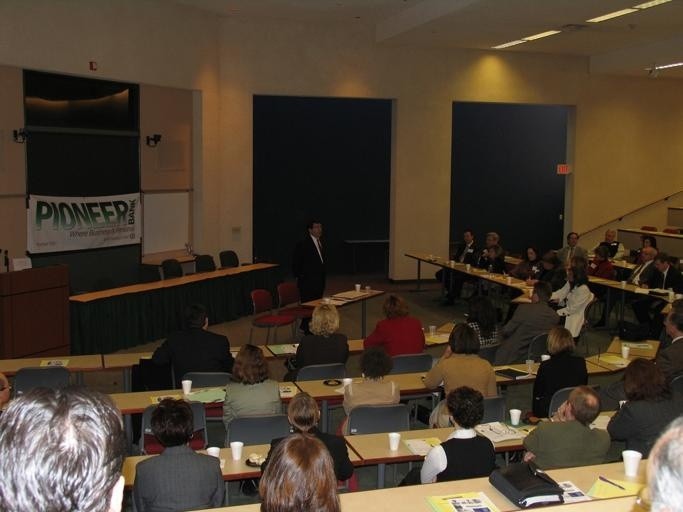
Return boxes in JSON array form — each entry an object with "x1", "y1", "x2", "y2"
[
  {"x1": 549, "y1": 385, "x2": 601, "y2": 419},
  {"x1": 248, "y1": 289, "x2": 297, "y2": 346},
  {"x1": 391, "y1": 353, "x2": 433, "y2": 373},
  {"x1": 183, "y1": 369, "x2": 233, "y2": 388},
  {"x1": 297, "y1": 362, "x2": 346, "y2": 381},
  {"x1": 224, "y1": 412, "x2": 292, "y2": 447},
  {"x1": 528, "y1": 332, "x2": 551, "y2": 361},
  {"x1": 481, "y1": 395, "x2": 507, "y2": 424},
  {"x1": 139, "y1": 263, "x2": 162, "y2": 283},
  {"x1": 349, "y1": 404, "x2": 409, "y2": 432},
  {"x1": 161, "y1": 259, "x2": 183, "y2": 280},
  {"x1": 11, "y1": 366, "x2": 71, "y2": 398},
  {"x1": 276, "y1": 283, "x2": 314, "y2": 341},
  {"x1": 139, "y1": 399, "x2": 208, "y2": 454},
  {"x1": 195, "y1": 254, "x2": 215, "y2": 273},
  {"x1": 220, "y1": 250, "x2": 240, "y2": 269}
]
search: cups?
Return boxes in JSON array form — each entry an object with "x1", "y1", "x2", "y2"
[
  {"x1": 450, "y1": 260, "x2": 455, "y2": 268},
  {"x1": 388, "y1": 433, "x2": 401, "y2": 451},
  {"x1": 324, "y1": 299, "x2": 330, "y2": 305},
  {"x1": 621, "y1": 281, "x2": 627, "y2": 288},
  {"x1": 230, "y1": 441, "x2": 243, "y2": 460},
  {"x1": 510, "y1": 409, "x2": 521, "y2": 425},
  {"x1": 343, "y1": 378, "x2": 352, "y2": 386},
  {"x1": 621, "y1": 261, "x2": 626, "y2": 267},
  {"x1": 356, "y1": 285, "x2": 361, "y2": 292},
  {"x1": 668, "y1": 288, "x2": 673, "y2": 293},
  {"x1": 526, "y1": 360, "x2": 534, "y2": 372},
  {"x1": 541, "y1": 355, "x2": 551, "y2": 362},
  {"x1": 622, "y1": 450, "x2": 642, "y2": 479},
  {"x1": 668, "y1": 292, "x2": 675, "y2": 300},
  {"x1": 465, "y1": 264, "x2": 470, "y2": 271},
  {"x1": 429, "y1": 325, "x2": 437, "y2": 337},
  {"x1": 366, "y1": 287, "x2": 370, "y2": 292},
  {"x1": 506, "y1": 277, "x2": 512, "y2": 284},
  {"x1": 428, "y1": 255, "x2": 432, "y2": 260},
  {"x1": 182, "y1": 380, "x2": 192, "y2": 394},
  {"x1": 207, "y1": 447, "x2": 220, "y2": 459},
  {"x1": 622, "y1": 347, "x2": 629, "y2": 359}
]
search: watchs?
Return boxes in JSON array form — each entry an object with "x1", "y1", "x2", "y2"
[{"x1": 550, "y1": 418, "x2": 555, "y2": 423}]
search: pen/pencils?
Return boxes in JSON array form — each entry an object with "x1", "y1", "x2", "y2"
[
  {"x1": 523, "y1": 428, "x2": 530, "y2": 434},
  {"x1": 607, "y1": 362, "x2": 627, "y2": 367},
  {"x1": 598, "y1": 474, "x2": 625, "y2": 491}
]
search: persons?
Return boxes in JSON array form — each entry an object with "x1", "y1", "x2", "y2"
[
  {"x1": 501, "y1": 247, "x2": 546, "y2": 327},
  {"x1": 523, "y1": 385, "x2": 611, "y2": 469},
  {"x1": 645, "y1": 237, "x2": 662, "y2": 256},
  {"x1": 440, "y1": 232, "x2": 502, "y2": 307},
  {"x1": 224, "y1": 343, "x2": 282, "y2": 441},
  {"x1": 153, "y1": 302, "x2": 231, "y2": 388},
  {"x1": 259, "y1": 392, "x2": 354, "y2": 481},
  {"x1": 363, "y1": 295, "x2": 427, "y2": 375},
  {"x1": 631, "y1": 253, "x2": 683, "y2": 341},
  {"x1": 549, "y1": 257, "x2": 588, "y2": 303},
  {"x1": 555, "y1": 232, "x2": 590, "y2": 287},
  {"x1": 435, "y1": 229, "x2": 480, "y2": 300},
  {"x1": 398, "y1": 386, "x2": 496, "y2": 486},
  {"x1": 588, "y1": 247, "x2": 617, "y2": 301},
  {"x1": 592, "y1": 229, "x2": 625, "y2": 301},
  {"x1": 468, "y1": 296, "x2": 498, "y2": 346},
  {"x1": 425, "y1": 323, "x2": 498, "y2": 429},
  {"x1": 607, "y1": 358, "x2": 683, "y2": 460},
  {"x1": 463, "y1": 246, "x2": 504, "y2": 318},
  {"x1": 295, "y1": 221, "x2": 327, "y2": 310},
  {"x1": 636, "y1": 416, "x2": 683, "y2": 511},
  {"x1": 497, "y1": 282, "x2": 560, "y2": 366},
  {"x1": 526, "y1": 252, "x2": 567, "y2": 292},
  {"x1": 132, "y1": 397, "x2": 225, "y2": 512},
  {"x1": 532, "y1": 327, "x2": 589, "y2": 418},
  {"x1": 258, "y1": 433, "x2": 341, "y2": 512},
  {"x1": 283, "y1": 304, "x2": 350, "y2": 382},
  {"x1": 557, "y1": 267, "x2": 595, "y2": 344},
  {"x1": 1, "y1": 385, "x2": 126, "y2": 512},
  {"x1": 655, "y1": 307, "x2": 683, "y2": 394},
  {"x1": 594, "y1": 246, "x2": 661, "y2": 337},
  {"x1": 624, "y1": 235, "x2": 647, "y2": 276}
]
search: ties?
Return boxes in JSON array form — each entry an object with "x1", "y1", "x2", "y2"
[
  {"x1": 317, "y1": 239, "x2": 324, "y2": 259},
  {"x1": 569, "y1": 247, "x2": 574, "y2": 261},
  {"x1": 660, "y1": 273, "x2": 665, "y2": 289},
  {"x1": 630, "y1": 263, "x2": 645, "y2": 284}
]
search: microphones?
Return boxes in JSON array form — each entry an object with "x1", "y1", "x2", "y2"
[{"x1": 4, "y1": 250, "x2": 8, "y2": 265}]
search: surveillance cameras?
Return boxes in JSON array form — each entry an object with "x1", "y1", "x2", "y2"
[
  {"x1": 146, "y1": 133, "x2": 161, "y2": 146},
  {"x1": 14, "y1": 127, "x2": 28, "y2": 142}
]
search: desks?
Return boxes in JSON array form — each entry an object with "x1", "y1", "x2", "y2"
[
  {"x1": 587, "y1": 353, "x2": 655, "y2": 374},
  {"x1": 494, "y1": 358, "x2": 610, "y2": 393},
  {"x1": 0, "y1": 353, "x2": 103, "y2": 391},
  {"x1": 294, "y1": 369, "x2": 444, "y2": 432},
  {"x1": 617, "y1": 225, "x2": 683, "y2": 259},
  {"x1": 424, "y1": 325, "x2": 454, "y2": 348},
  {"x1": 667, "y1": 207, "x2": 683, "y2": 225},
  {"x1": 587, "y1": 250, "x2": 637, "y2": 270},
  {"x1": 68, "y1": 262, "x2": 279, "y2": 356},
  {"x1": 104, "y1": 343, "x2": 274, "y2": 392},
  {"x1": 108, "y1": 381, "x2": 304, "y2": 456},
  {"x1": 345, "y1": 424, "x2": 537, "y2": 488},
  {"x1": 121, "y1": 437, "x2": 365, "y2": 506},
  {"x1": 301, "y1": 289, "x2": 385, "y2": 339},
  {"x1": 607, "y1": 336, "x2": 661, "y2": 357},
  {"x1": 587, "y1": 274, "x2": 683, "y2": 332},
  {"x1": 405, "y1": 248, "x2": 538, "y2": 305},
  {"x1": 266, "y1": 332, "x2": 434, "y2": 357},
  {"x1": 337, "y1": 455, "x2": 652, "y2": 512}
]
